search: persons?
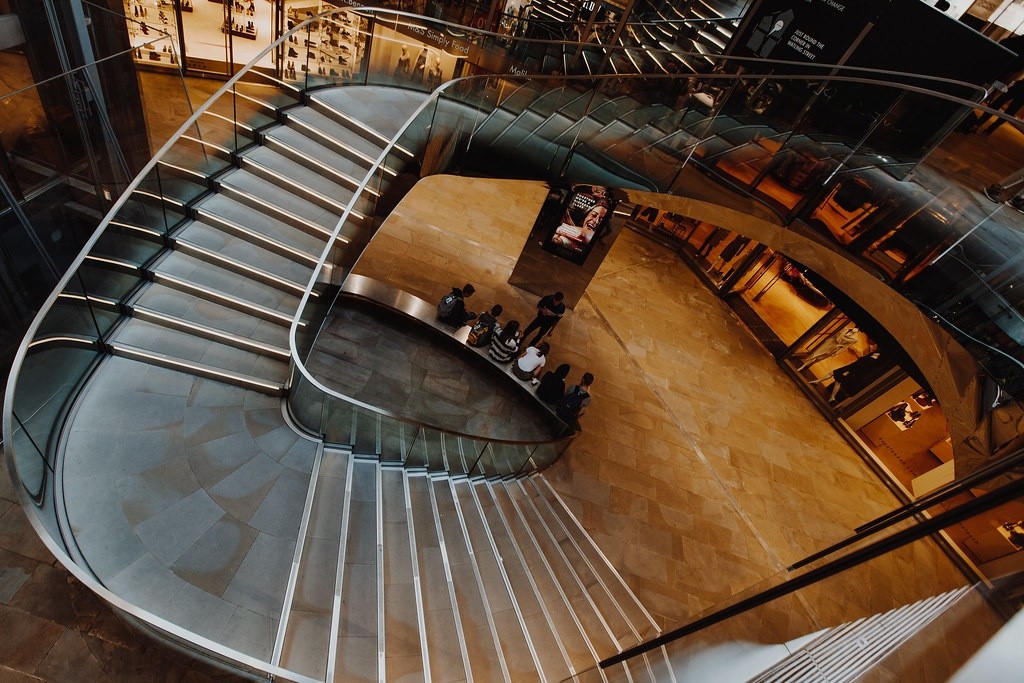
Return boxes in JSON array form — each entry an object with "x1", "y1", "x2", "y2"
[
  {"x1": 694, "y1": 228, "x2": 757, "y2": 289},
  {"x1": 468, "y1": 304, "x2": 502, "y2": 347},
  {"x1": 437, "y1": 284, "x2": 477, "y2": 326},
  {"x1": 536, "y1": 364, "x2": 570, "y2": 406},
  {"x1": 520, "y1": 292, "x2": 566, "y2": 347},
  {"x1": 393, "y1": 44, "x2": 442, "y2": 89},
  {"x1": 553, "y1": 204, "x2": 609, "y2": 252},
  {"x1": 512, "y1": 342, "x2": 550, "y2": 386},
  {"x1": 797, "y1": 328, "x2": 859, "y2": 372},
  {"x1": 489, "y1": 320, "x2": 523, "y2": 364},
  {"x1": 809, "y1": 352, "x2": 880, "y2": 402},
  {"x1": 557, "y1": 372, "x2": 594, "y2": 433}
]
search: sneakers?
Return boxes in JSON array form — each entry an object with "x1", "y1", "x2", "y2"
[
  {"x1": 983, "y1": 182, "x2": 1005, "y2": 204},
  {"x1": 1005, "y1": 194, "x2": 1024, "y2": 214}
]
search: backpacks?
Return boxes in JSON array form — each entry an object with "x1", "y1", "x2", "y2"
[
  {"x1": 558, "y1": 385, "x2": 590, "y2": 422},
  {"x1": 468, "y1": 319, "x2": 494, "y2": 345},
  {"x1": 437, "y1": 292, "x2": 464, "y2": 318}
]
